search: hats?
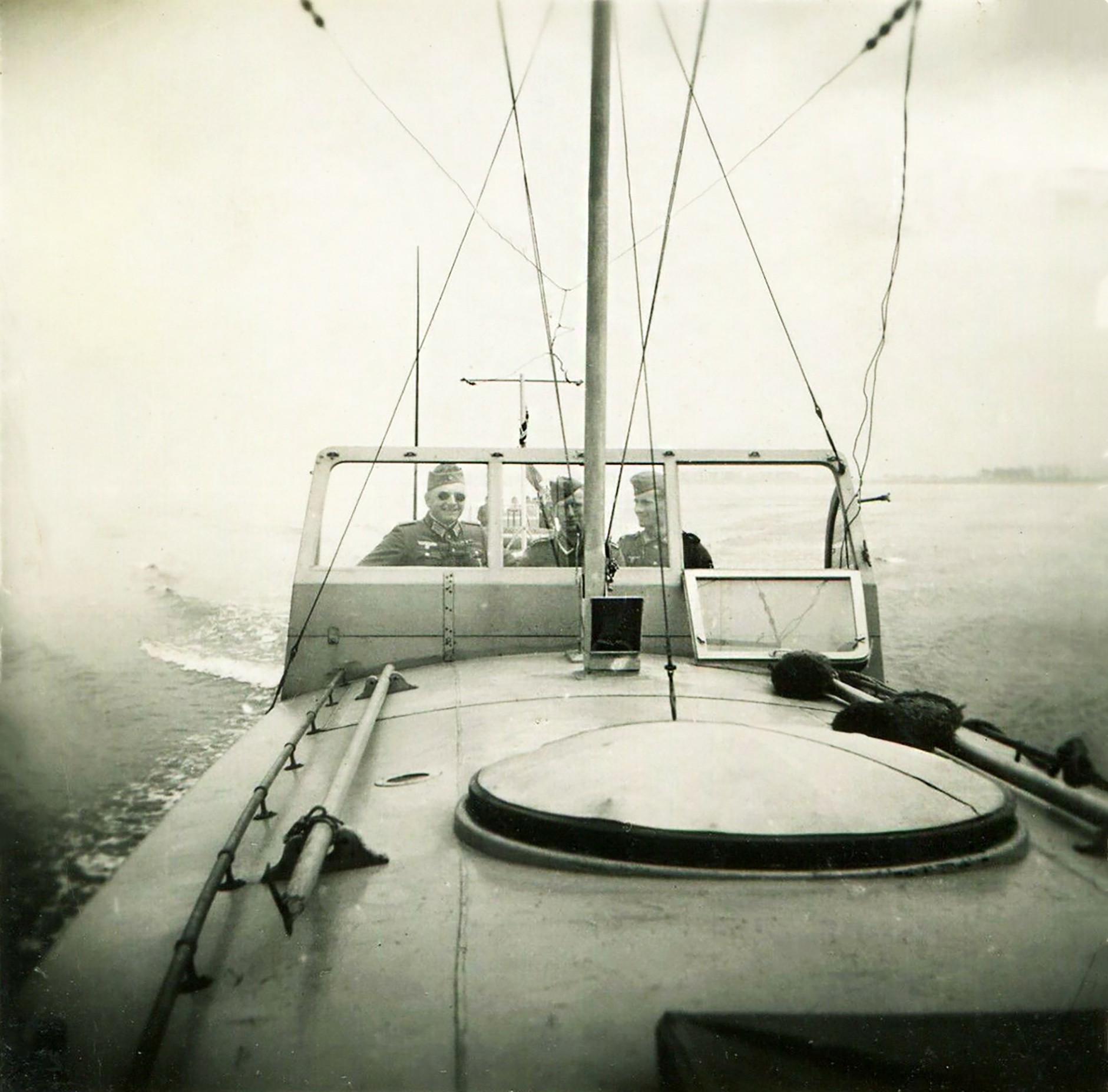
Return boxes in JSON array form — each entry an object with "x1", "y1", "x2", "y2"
[
  {"x1": 428, "y1": 463, "x2": 466, "y2": 490},
  {"x1": 549, "y1": 476, "x2": 583, "y2": 504},
  {"x1": 630, "y1": 470, "x2": 664, "y2": 496}
]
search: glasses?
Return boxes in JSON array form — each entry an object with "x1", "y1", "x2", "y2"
[{"x1": 431, "y1": 492, "x2": 465, "y2": 502}]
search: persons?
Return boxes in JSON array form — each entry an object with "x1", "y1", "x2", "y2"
[
  {"x1": 617, "y1": 472, "x2": 716, "y2": 569},
  {"x1": 355, "y1": 463, "x2": 515, "y2": 567},
  {"x1": 475, "y1": 494, "x2": 559, "y2": 529},
  {"x1": 515, "y1": 476, "x2": 628, "y2": 567}
]
